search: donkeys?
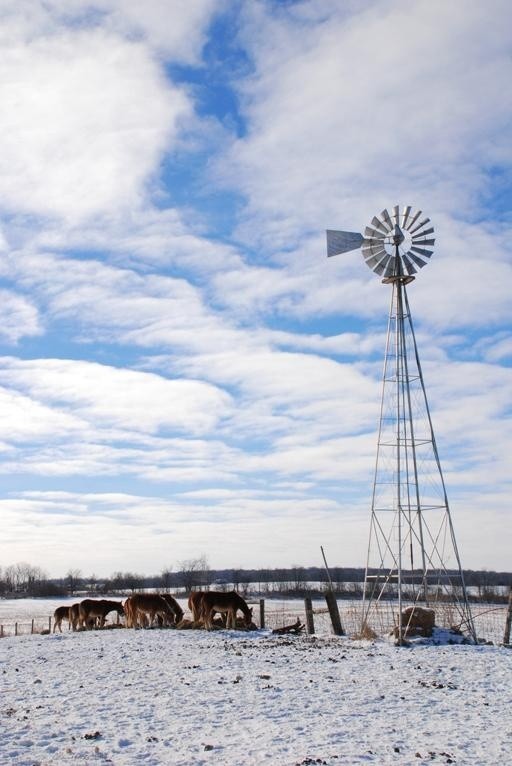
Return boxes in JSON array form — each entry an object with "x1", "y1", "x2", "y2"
[
  {"x1": 188, "y1": 590, "x2": 253, "y2": 631},
  {"x1": 69, "y1": 603, "x2": 108, "y2": 631},
  {"x1": 124, "y1": 594, "x2": 185, "y2": 629},
  {"x1": 52, "y1": 606, "x2": 95, "y2": 634},
  {"x1": 79, "y1": 599, "x2": 125, "y2": 630}
]
